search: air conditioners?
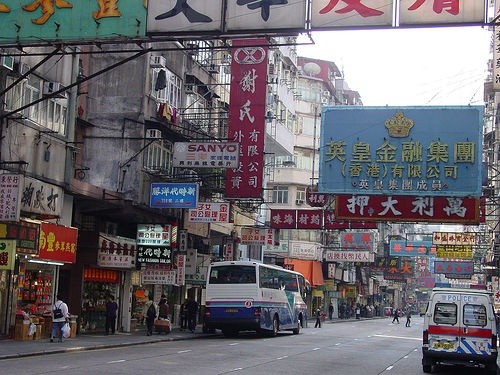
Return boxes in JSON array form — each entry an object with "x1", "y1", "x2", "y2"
[
  {"x1": 43, "y1": 83, "x2": 67, "y2": 98},
  {"x1": 285, "y1": 155, "x2": 296, "y2": 166},
  {"x1": 322, "y1": 91, "x2": 334, "y2": 103},
  {"x1": 295, "y1": 198, "x2": 304, "y2": 205},
  {"x1": 182, "y1": 170, "x2": 198, "y2": 182},
  {"x1": 9, "y1": 63, "x2": 31, "y2": 79},
  {"x1": 187, "y1": 43, "x2": 200, "y2": 56},
  {"x1": 150, "y1": 129, "x2": 161, "y2": 138},
  {"x1": 186, "y1": 83, "x2": 199, "y2": 94},
  {"x1": 222, "y1": 54, "x2": 232, "y2": 64},
  {"x1": 0, "y1": 53, "x2": 14, "y2": 71},
  {"x1": 151, "y1": 56, "x2": 166, "y2": 68},
  {"x1": 343, "y1": 99, "x2": 347, "y2": 105},
  {"x1": 208, "y1": 64, "x2": 221, "y2": 73},
  {"x1": 103, "y1": 220, "x2": 119, "y2": 234}
]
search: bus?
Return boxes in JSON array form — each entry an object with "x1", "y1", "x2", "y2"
[{"x1": 203, "y1": 259, "x2": 311, "y2": 338}]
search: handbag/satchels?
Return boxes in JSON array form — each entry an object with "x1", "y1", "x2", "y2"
[{"x1": 61, "y1": 323, "x2": 71, "y2": 338}]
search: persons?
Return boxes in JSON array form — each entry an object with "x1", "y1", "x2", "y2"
[
  {"x1": 179, "y1": 297, "x2": 198, "y2": 332},
  {"x1": 340, "y1": 302, "x2": 364, "y2": 319},
  {"x1": 315, "y1": 306, "x2": 322, "y2": 328},
  {"x1": 105, "y1": 296, "x2": 117, "y2": 335},
  {"x1": 157, "y1": 294, "x2": 169, "y2": 320},
  {"x1": 392, "y1": 309, "x2": 400, "y2": 324},
  {"x1": 329, "y1": 303, "x2": 333, "y2": 320},
  {"x1": 145, "y1": 295, "x2": 157, "y2": 335},
  {"x1": 50, "y1": 295, "x2": 70, "y2": 342},
  {"x1": 406, "y1": 314, "x2": 411, "y2": 327}
]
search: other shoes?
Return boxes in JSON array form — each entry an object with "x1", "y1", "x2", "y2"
[
  {"x1": 105, "y1": 333, "x2": 109, "y2": 335},
  {"x1": 59, "y1": 341, "x2": 63, "y2": 343},
  {"x1": 147, "y1": 332, "x2": 152, "y2": 336},
  {"x1": 50, "y1": 339, "x2": 53, "y2": 343}
]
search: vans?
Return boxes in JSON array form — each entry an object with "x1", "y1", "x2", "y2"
[{"x1": 420, "y1": 286, "x2": 500, "y2": 375}]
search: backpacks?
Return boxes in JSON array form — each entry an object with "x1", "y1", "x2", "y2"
[
  {"x1": 147, "y1": 303, "x2": 156, "y2": 317},
  {"x1": 53, "y1": 303, "x2": 63, "y2": 319}
]
